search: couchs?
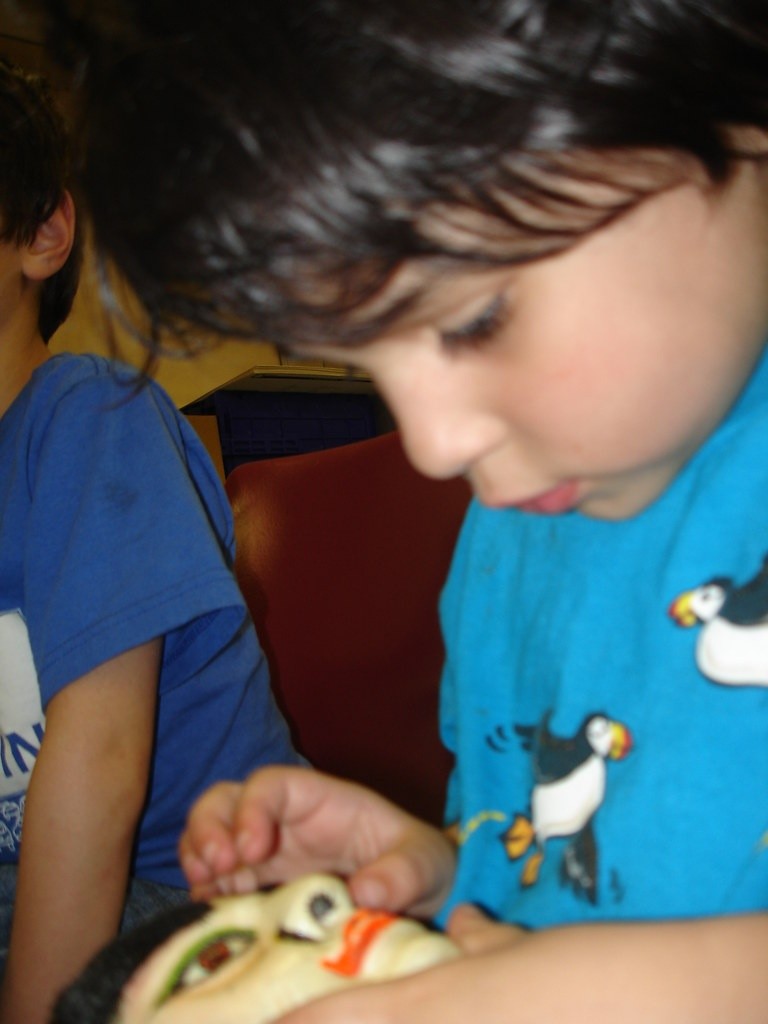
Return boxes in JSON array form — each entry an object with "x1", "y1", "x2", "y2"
[{"x1": 222, "y1": 426, "x2": 475, "y2": 833}]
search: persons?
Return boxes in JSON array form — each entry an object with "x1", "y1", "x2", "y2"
[
  {"x1": 53, "y1": 874, "x2": 460, "y2": 1024},
  {"x1": 75, "y1": 0, "x2": 768, "y2": 1024},
  {"x1": 0, "y1": 76, "x2": 303, "y2": 1024}
]
